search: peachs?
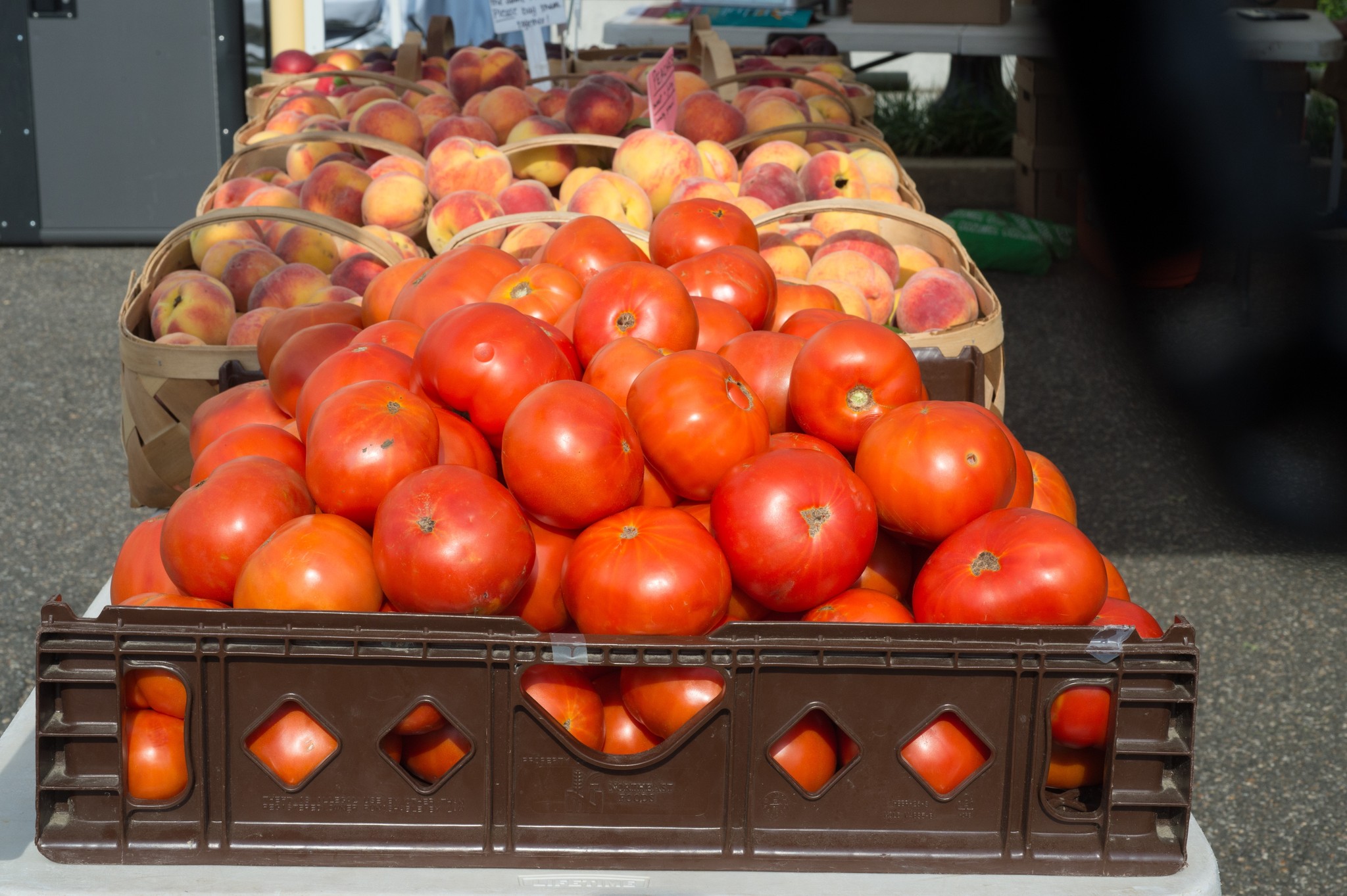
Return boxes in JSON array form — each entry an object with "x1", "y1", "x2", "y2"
[{"x1": 143, "y1": 46, "x2": 980, "y2": 346}]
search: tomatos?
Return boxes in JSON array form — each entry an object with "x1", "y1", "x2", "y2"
[{"x1": 108, "y1": 198, "x2": 1163, "y2": 798}]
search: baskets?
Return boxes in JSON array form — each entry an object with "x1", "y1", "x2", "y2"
[{"x1": 35, "y1": 596, "x2": 1201, "y2": 877}]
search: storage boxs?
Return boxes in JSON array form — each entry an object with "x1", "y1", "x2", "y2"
[
  {"x1": 31, "y1": 589, "x2": 1203, "y2": 875},
  {"x1": 1012, "y1": 58, "x2": 1076, "y2": 225}
]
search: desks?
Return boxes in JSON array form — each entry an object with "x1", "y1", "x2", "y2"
[
  {"x1": 0, "y1": 573, "x2": 1223, "y2": 896},
  {"x1": 597, "y1": 8, "x2": 1345, "y2": 62}
]
